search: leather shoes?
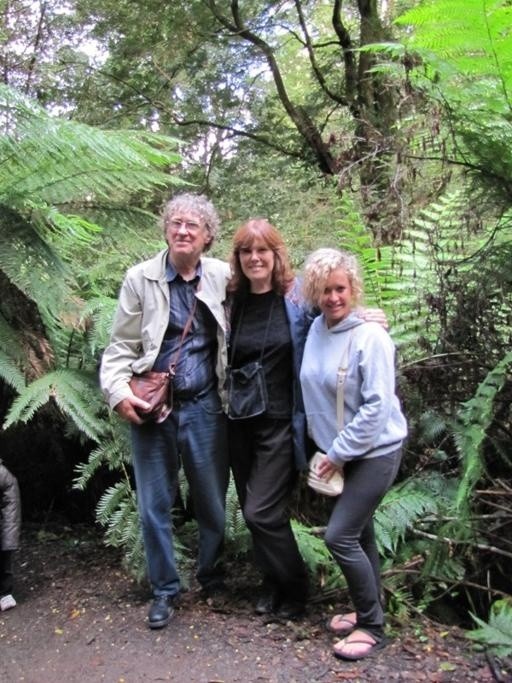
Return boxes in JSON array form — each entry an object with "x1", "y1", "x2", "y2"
[
  {"x1": 257, "y1": 596, "x2": 306, "y2": 617},
  {"x1": 148, "y1": 594, "x2": 176, "y2": 628},
  {"x1": 202, "y1": 583, "x2": 234, "y2": 610}
]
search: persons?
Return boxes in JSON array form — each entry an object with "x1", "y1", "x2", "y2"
[
  {"x1": 0, "y1": 462, "x2": 22, "y2": 612},
  {"x1": 222, "y1": 217, "x2": 389, "y2": 618},
  {"x1": 292, "y1": 247, "x2": 411, "y2": 659},
  {"x1": 95, "y1": 189, "x2": 236, "y2": 631}
]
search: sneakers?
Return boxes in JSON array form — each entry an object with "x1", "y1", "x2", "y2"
[{"x1": 1, "y1": 594, "x2": 16, "y2": 611}]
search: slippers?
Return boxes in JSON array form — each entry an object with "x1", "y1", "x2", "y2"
[
  {"x1": 326, "y1": 612, "x2": 358, "y2": 632},
  {"x1": 335, "y1": 629, "x2": 387, "y2": 659}
]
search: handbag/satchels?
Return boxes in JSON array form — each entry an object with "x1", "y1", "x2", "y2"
[
  {"x1": 221, "y1": 363, "x2": 268, "y2": 421},
  {"x1": 129, "y1": 370, "x2": 173, "y2": 424},
  {"x1": 309, "y1": 451, "x2": 344, "y2": 497}
]
